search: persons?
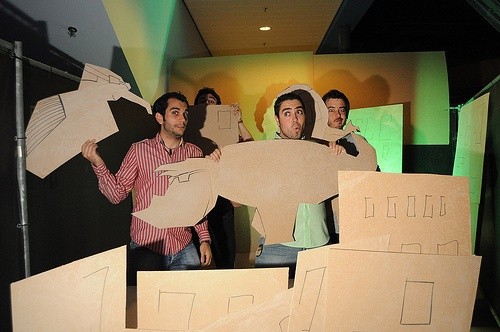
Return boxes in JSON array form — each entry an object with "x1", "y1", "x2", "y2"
[
  {"x1": 194, "y1": 88, "x2": 255, "y2": 268},
  {"x1": 204, "y1": 93, "x2": 347, "y2": 279},
  {"x1": 316, "y1": 90, "x2": 381, "y2": 244},
  {"x1": 81, "y1": 91, "x2": 212, "y2": 271}
]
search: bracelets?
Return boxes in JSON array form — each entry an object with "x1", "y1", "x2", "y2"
[
  {"x1": 201, "y1": 240, "x2": 211, "y2": 246},
  {"x1": 238, "y1": 120, "x2": 243, "y2": 123}
]
